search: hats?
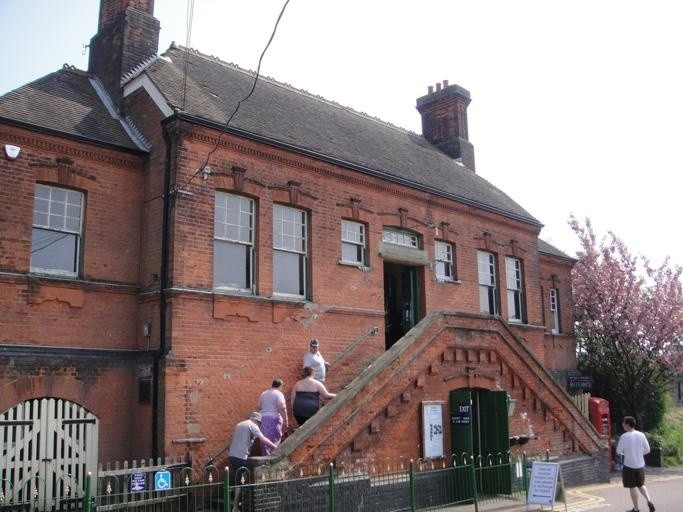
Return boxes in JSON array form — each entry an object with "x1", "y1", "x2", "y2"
[
  {"x1": 248, "y1": 411, "x2": 263, "y2": 423},
  {"x1": 309, "y1": 337, "x2": 320, "y2": 348}
]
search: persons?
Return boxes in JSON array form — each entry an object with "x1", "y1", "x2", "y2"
[
  {"x1": 257, "y1": 379, "x2": 288, "y2": 456},
  {"x1": 303, "y1": 338, "x2": 331, "y2": 385},
  {"x1": 290, "y1": 367, "x2": 336, "y2": 426},
  {"x1": 228, "y1": 411, "x2": 277, "y2": 512},
  {"x1": 615, "y1": 416, "x2": 655, "y2": 512}
]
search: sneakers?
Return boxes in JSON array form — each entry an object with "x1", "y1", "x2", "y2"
[
  {"x1": 647, "y1": 503, "x2": 655, "y2": 512},
  {"x1": 627, "y1": 507, "x2": 640, "y2": 512}
]
